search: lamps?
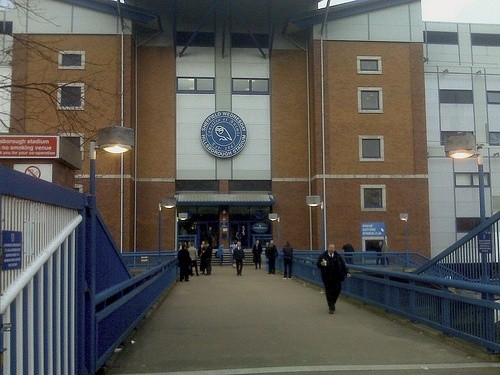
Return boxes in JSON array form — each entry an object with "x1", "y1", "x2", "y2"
[
  {"x1": 179, "y1": 213, "x2": 188, "y2": 220},
  {"x1": 445, "y1": 132, "x2": 476, "y2": 159},
  {"x1": 162, "y1": 198, "x2": 176, "y2": 209},
  {"x1": 268, "y1": 214, "x2": 277, "y2": 221},
  {"x1": 98, "y1": 126, "x2": 135, "y2": 153},
  {"x1": 306, "y1": 196, "x2": 321, "y2": 206}
]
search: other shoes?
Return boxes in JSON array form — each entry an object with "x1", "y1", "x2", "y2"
[{"x1": 329, "y1": 308, "x2": 334, "y2": 314}]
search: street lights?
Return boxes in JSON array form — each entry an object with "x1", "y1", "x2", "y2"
[
  {"x1": 268, "y1": 213, "x2": 281, "y2": 252},
  {"x1": 175, "y1": 212, "x2": 188, "y2": 255},
  {"x1": 399, "y1": 212, "x2": 409, "y2": 252},
  {"x1": 443, "y1": 133, "x2": 489, "y2": 285},
  {"x1": 306, "y1": 195, "x2": 326, "y2": 251},
  {"x1": 158, "y1": 197, "x2": 176, "y2": 266},
  {"x1": 88, "y1": 125, "x2": 135, "y2": 375}
]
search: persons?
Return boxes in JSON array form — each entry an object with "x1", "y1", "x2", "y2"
[
  {"x1": 317, "y1": 242, "x2": 351, "y2": 314},
  {"x1": 179, "y1": 225, "x2": 293, "y2": 281},
  {"x1": 376, "y1": 242, "x2": 390, "y2": 265},
  {"x1": 342, "y1": 242, "x2": 354, "y2": 265}
]
